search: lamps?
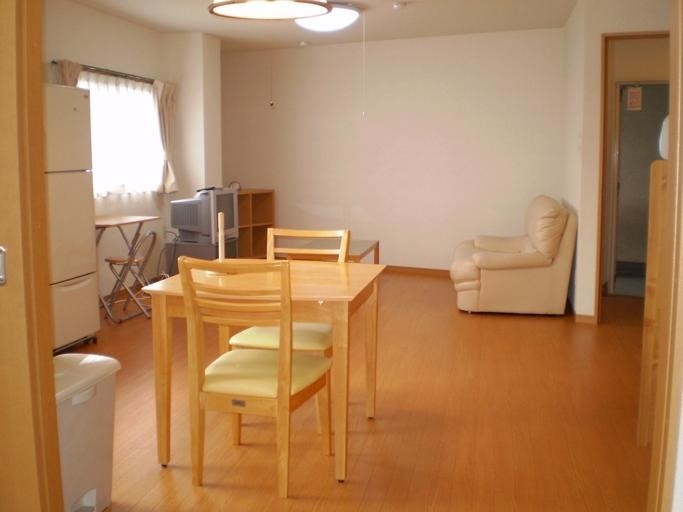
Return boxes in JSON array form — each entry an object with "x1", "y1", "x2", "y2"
[{"x1": 208, "y1": 0, "x2": 369, "y2": 35}]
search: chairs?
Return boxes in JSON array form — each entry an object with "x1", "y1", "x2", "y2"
[
  {"x1": 176, "y1": 252, "x2": 334, "y2": 496},
  {"x1": 228, "y1": 225, "x2": 353, "y2": 449},
  {"x1": 103, "y1": 229, "x2": 161, "y2": 322},
  {"x1": 449, "y1": 194, "x2": 578, "y2": 317}
]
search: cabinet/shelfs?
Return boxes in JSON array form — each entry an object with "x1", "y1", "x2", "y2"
[{"x1": 238, "y1": 188, "x2": 276, "y2": 260}]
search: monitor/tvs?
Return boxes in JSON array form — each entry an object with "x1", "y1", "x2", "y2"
[{"x1": 170, "y1": 188, "x2": 239, "y2": 244}]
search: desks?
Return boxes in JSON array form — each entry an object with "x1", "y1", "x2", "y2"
[
  {"x1": 140, "y1": 256, "x2": 387, "y2": 485},
  {"x1": 95, "y1": 215, "x2": 162, "y2": 323}
]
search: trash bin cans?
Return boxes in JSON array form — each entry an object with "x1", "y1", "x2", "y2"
[{"x1": 53, "y1": 354, "x2": 121, "y2": 512}]
